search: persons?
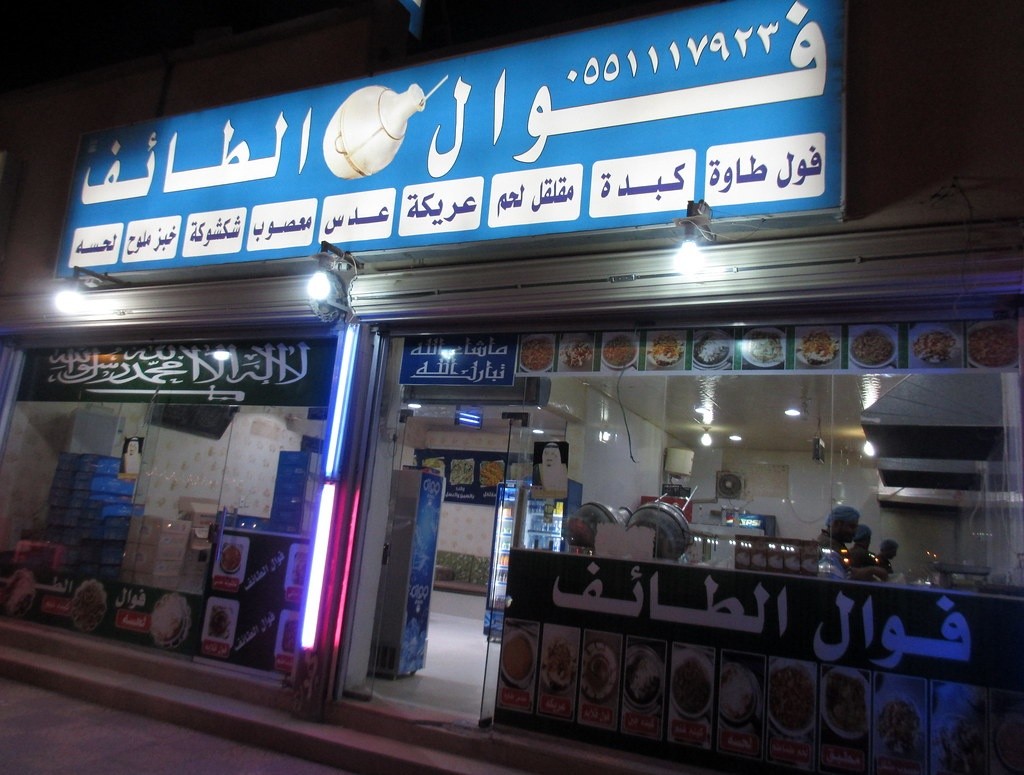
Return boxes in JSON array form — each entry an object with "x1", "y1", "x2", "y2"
[
  {"x1": 848, "y1": 524, "x2": 878, "y2": 566},
  {"x1": 818, "y1": 506, "x2": 888, "y2": 579},
  {"x1": 878, "y1": 539, "x2": 898, "y2": 573}
]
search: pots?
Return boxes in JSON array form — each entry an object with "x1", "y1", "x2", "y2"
[
  {"x1": 626, "y1": 501, "x2": 695, "y2": 560},
  {"x1": 565, "y1": 502, "x2": 631, "y2": 547}
]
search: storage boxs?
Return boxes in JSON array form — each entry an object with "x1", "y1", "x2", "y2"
[
  {"x1": 217, "y1": 451, "x2": 322, "y2": 535},
  {"x1": 44, "y1": 453, "x2": 220, "y2": 595}
]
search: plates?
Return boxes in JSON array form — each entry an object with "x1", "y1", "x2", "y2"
[
  {"x1": 520, "y1": 334, "x2": 555, "y2": 372},
  {"x1": 540, "y1": 651, "x2": 576, "y2": 693},
  {"x1": 0, "y1": 534, "x2": 243, "y2": 650},
  {"x1": 501, "y1": 630, "x2": 536, "y2": 689},
  {"x1": 743, "y1": 328, "x2": 785, "y2": 368},
  {"x1": 913, "y1": 328, "x2": 959, "y2": 364},
  {"x1": 558, "y1": 333, "x2": 593, "y2": 370},
  {"x1": 671, "y1": 649, "x2": 714, "y2": 718},
  {"x1": 966, "y1": 320, "x2": 1019, "y2": 369},
  {"x1": 821, "y1": 667, "x2": 1024, "y2": 775},
  {"x1": 693, "y1": 329, "x2": 732, "y2": 370},
  {"x1": 720, "y1": 664, "x2": 760, "y2": 723},
  {"x1": 768, "y1": 659, "x2": 816, "y2": 736},
  {"x1": 602, "y1": 333, "x2": 640, "y2": 369},
  {"x1": 623, "y1": 645, "x2": 664, "y2": 714},
  {"x1": 581, "y1": 641, "x2": 620, "y2": 704},
  {"x1": 646, "y1": 334, "x2": 683, "y2": 367},
  {"x1": 848, "y1": 325, "x2": 899, "y2": 368},
  {"x1": 796, "y1": 333, "x2": 839, "y2": 367}
]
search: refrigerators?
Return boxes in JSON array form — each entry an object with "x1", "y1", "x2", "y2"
[
  {"x1": 379, "y1": 469, "x2": 446, "y2": 680},
  {"x1": 484, "y1": 478, "x2": 584, "y2": 642}
]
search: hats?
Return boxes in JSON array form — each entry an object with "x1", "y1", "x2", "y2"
[
  {"x1": 828, "y1": 506, "x2": 860, "y2": 524},
  {"x1": 852, "y1": 524, "x2": 872, "y2": 542},
  {"x1": 879, "y1": 539, "x2": 898, "y2": 549}
]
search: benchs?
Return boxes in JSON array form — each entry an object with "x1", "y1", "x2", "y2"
[{"x1": 433, "y1": 551, "x2": 492, "y2": 620}]
